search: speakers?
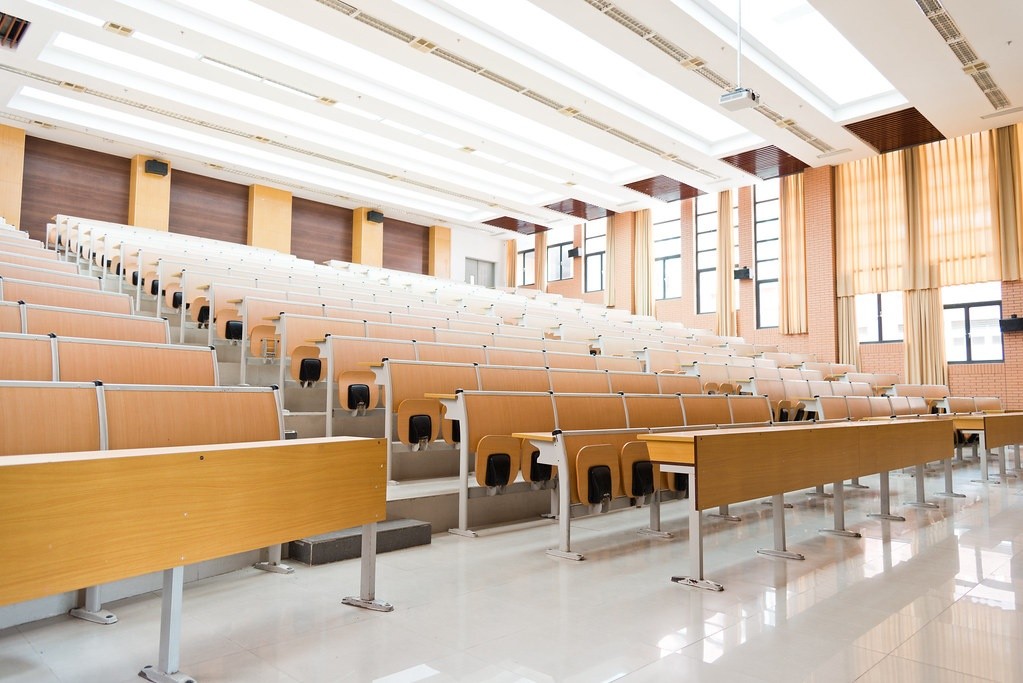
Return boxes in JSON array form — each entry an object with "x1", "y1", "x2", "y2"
[
  {"x1": 568, "y1": 248, "x2": 582, "y2": 257},
  {"x1": 145, "y1": 159, "x2": 168, "y2": 177},
  {"x1": 1000, "y1": 318, "x2": 1023, "y2": 333},
  {"x1": 734, "y1": 268, "x2": 752, "y2": 279},
  {"x1": 367, "y1": 211, "x2": 384, "y2": 223}
]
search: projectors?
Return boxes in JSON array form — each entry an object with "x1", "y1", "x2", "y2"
[{"x1": 719, "y1": 89, "x2": 760, "y2": 112}]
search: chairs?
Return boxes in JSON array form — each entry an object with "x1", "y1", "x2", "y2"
[
  {"x1": 339, "y1": 371, "x2": 379, "y2": 418},
  {"x1": 792, "y1": 402, "x2": 808, "y2": 421},
  {"x1": 805, "y1": 410, "x2": 820, "y2": 420},
  {"x1": 476, "y1": 435, "x2": 521, "y2": 497},
  {"x1": 776, "y1": 400, "x2": 791, "y2": 422},
  {"x1": 48, "y1": 226, "x2": 60, "y2": 244},
  {"x1": 736, "y1": 385, "x2": 751, "y2": 395},
  {"x1": 191, "y1": 297, "x2": 210, "y2": 329},
  {"x1": 165, "y1": 282, "x2": 189, "y2": 314},
  {"x1": 61, "y1": 230, "x2": 70, "y2": 248},
  {"x1": 125, "y1": 262, "x2": 144, "y2": 286},
  {"x1": 250, "y1": 325, "x2": 282, "y2": 364},
  {"x1": 589, "y1": 344, "x2": 600, "y2": 355},
  {"x1": 620, "y1": 441, "x2": 660, "y2": 507},
  {"x1": 703, "y1": 382, "x2": 718, "y2": 394},
  {"x1": 575, "y1": 443, "x2": 620, "y2": 514},
  {"x1": 719, "y1": 384, "x2": 733, "y2": 395},
  {"x1": 83, "y1": 242, "x2": 97, "y2": 261},
  {"x1": 110, "y1": 256, "x2": 121, "y2": 274},
  {"x1": 216, "y1": 309, "x2": 243, "y2": 345},
  {"x1": 667, "y1": 472, "x2": 688, "y2": 499},
  {"x1": 519, "y1": 438, "x2": 558, "y2": 491},
  {"x1": 290, "y1": 346, "x2": 327, "y2": 389},
  {"x1": 95, "y1": 247, "x2": 111, "y2": 267},
  {"x1": 441, "y1": 406, "x2": 460, "y2": 449},
  {"x1": 70, "y1": 233, "x2": 83, "y2": 254},
  {"x1": 398, "y1": 399, "x2": 440, "y2": 452},
  {"x1": 144, "y1": 271, "x2": 166, "y2": 300}
]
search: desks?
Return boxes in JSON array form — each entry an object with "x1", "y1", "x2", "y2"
[{"x1": 0, "y1": 214, "x2": 1023, "y2": 683}]
